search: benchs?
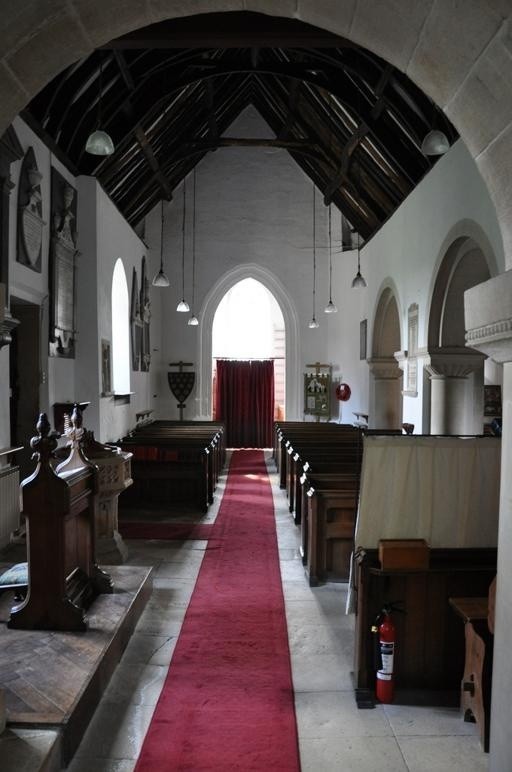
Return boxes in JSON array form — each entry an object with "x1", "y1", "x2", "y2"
[
  {"x1": 111, "y1": 421, "x2": 225, "y2": 513},
  {"x1": 274, "y1": 420, "x2": 369, "y2": 586}
]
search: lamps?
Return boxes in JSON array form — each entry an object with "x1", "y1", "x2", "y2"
[
  {"x1": 151, "y1": 166, "x2": 199, "y2": 326},
  {"x1": 86, "y1": 56, "x2": 114, "y2": 158},
  {"x1": 422, "y1": 99, "x2": 449, "y2": 156},
  {"x1": 309, "y1": 93, "x2": 367, "y2": 329}
]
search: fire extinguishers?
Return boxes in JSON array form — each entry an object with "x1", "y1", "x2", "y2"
[{"x1": 371, "y1": 602, "x2": 409, "y2": 704}]
